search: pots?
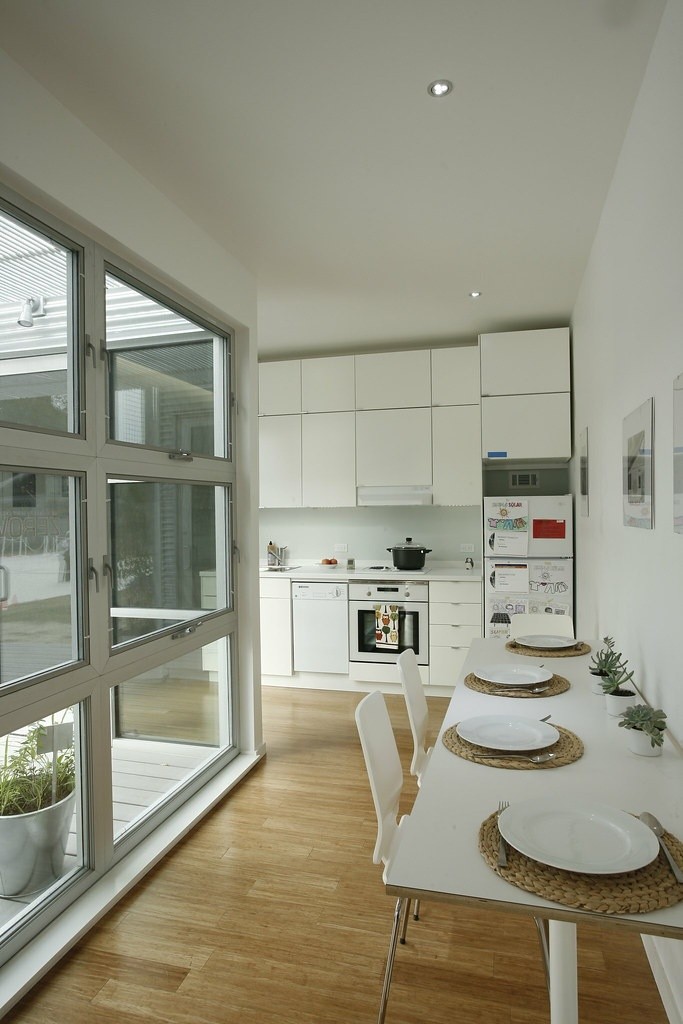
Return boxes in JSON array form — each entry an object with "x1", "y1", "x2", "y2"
[{"x1": 386, "y1": 537, "x2": 433, "y2": 569}]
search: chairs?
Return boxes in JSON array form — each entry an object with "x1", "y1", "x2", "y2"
[
  {"x1": 396, "y1": 648, "x2": 433, "y2": 788},
  {"x1": 510, "y1": 614, "x2": 574, "y2": 638},
  {"x1": 355, "y1": 690, "x2": 550, "y2": 1024}
]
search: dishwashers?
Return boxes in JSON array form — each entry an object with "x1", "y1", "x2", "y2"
[{"x1": 292, "y1": 583, "x2": 348, "y2": 674}]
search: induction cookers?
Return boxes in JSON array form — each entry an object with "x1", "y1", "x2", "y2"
[{"x1": 360, "y1": 563, "x2": 432, "y2": 575}]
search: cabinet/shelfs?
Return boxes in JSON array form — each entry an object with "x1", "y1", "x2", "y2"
[
  {"x1": 259, "y1": 327, "x2": 572, "y2": 510},
  {"x1": 201, "y1": 576, "x2": 482, "y2": 698}
]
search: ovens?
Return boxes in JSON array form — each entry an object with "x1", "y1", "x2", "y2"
[{"x1": 348, "y1": 580, "x2": 430, "y2": 685}]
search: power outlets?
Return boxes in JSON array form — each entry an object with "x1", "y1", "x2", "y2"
[
  {"x1": 460, "y1": 544, "x2": 475, "y2": 552},
  {"x1": 335, "y1": 544, "x2": 348, "y2": 552}
]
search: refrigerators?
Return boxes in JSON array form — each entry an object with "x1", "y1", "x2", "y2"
[{"x1": 483, "y1": 495, "x2": 575, "y2": 640}]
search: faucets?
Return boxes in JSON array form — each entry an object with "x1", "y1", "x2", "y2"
[{"x1": 268, "y1": 546, "x2": 287, "y2": 566}]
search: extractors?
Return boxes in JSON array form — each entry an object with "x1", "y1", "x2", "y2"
[{"x1": 357, "y1": 495, "x2": 433, "y2": 506}]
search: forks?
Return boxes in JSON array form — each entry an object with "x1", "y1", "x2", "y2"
[
  {"x1": 540, "y1": 664, "x2": 544, "y2": 668},
  {"x1": 497, "y1": 802, "x2": 512, "y2": 868},
  {"x1": 538, "y1": 714, "x2": 551, "y2": 722}
]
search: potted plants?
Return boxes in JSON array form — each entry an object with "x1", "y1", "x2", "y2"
[
  {"x1": 617, "y1": 704, "x2": 667, "y2": 756},
  {"x1": 598, "y1": 667, "x2": 637, "y2": 715},
  {"x1": 588, "y1": 635, "x2": 629, "y2": 695},
  {"x1": 0, "y1": 706, "x2": 76, "y2": 898}
]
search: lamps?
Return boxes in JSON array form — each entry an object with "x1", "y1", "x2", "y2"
[{"x1": 17, "y1": 296, "x2": 46, "y2": 328}]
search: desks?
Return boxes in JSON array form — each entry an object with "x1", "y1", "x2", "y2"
[{"x1": 385, "y1": 635, "x2": 683, "y2": 1024}]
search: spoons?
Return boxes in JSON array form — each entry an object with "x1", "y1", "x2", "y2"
[
  {"x1": 489, "y1": 687, "x2": 549, "y2": 693},
  {"x1": 475, "y1": 753, "x2": 556, "y2": 763},
  {"x1": 639, "y1": 812, "x2": 683, "y2": 884}
]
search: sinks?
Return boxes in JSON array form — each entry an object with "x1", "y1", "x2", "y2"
[{"x1": 259, "y1": 567, "x2": 299, "y2": 572}]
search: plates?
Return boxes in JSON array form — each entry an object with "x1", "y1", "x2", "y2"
[
  {"x1": 515, "y1": 635, "x2": 577, "y2": 650},
  {"x1": 457, "y1": 714, "x2": 559, "y2": 750},
  {"x1": 500, "y1": 800, "x2": 659, "y2": 874},
  {"x1": 261, "y1": 565, "x2": 300, "y2": 569},
  {"x1": 474, "y1": 665, "x2": 553, "y2": 684},
  {"x1": 316, "y1": 563, "x2": 337, "y2": 568}
]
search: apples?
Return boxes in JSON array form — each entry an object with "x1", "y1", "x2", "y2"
[{"x1": 322, "y1": 559, "x2": 337, "y2": 564}]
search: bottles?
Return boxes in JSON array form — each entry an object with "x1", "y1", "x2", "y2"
[{"x1": 267, "y1": 541, "x2": 278, "y2": 565}]
